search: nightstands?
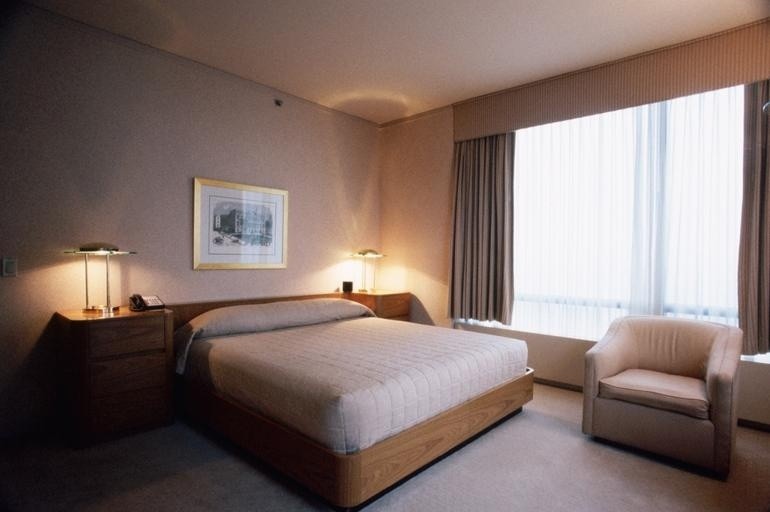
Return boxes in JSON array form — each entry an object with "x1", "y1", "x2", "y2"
[
  {"x1": 53, "y1": 304, "x2": 173, "y2": 436},
  {"x1": 341, "y1": 291, "x2": 411, "y2": 325}
]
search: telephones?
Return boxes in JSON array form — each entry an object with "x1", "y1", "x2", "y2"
[{"x1": 129, "y1": 294, "x2": 166, "y2": 312}]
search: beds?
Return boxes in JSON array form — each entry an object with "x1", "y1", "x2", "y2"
[{"x1": 168, "y1": 294, "x2": 534, "y2": 512}]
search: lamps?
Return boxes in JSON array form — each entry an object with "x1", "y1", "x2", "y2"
[
  {"x1": 352, "y1": 249, "x2": 387, "y2": 292},
  {"x1": 61, "y1": 243, "x2": 139, "y2": 312}
]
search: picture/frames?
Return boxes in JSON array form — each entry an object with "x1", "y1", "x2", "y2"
[{"x1": 191, "y1": 177, "x2": 288, "y2": 270}]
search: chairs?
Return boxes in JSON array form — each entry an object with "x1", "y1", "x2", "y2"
[{"x1": 583, "y1": 315, "x2": 745, "y2": 483}]
